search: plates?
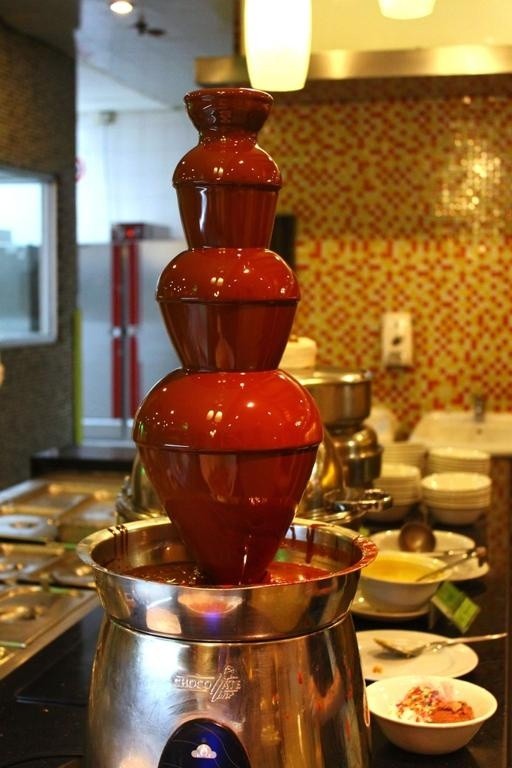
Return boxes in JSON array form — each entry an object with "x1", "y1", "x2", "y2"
[
  {"x1": 350, "y1": 590, "x2": 430, "y2": 621},
  {"x1": 443, "y1": 554, "x2": 490, "y2": 588},
  {"x1": 369, "y1": 529, "x2": 476, "y2": 561},
  {"x1": 356, "y1": 629, "x2": 480, "y2": 681}
]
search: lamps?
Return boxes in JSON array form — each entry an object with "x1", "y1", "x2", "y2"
[
  {"x1": 107, "y1": 0, "x2": 133, "y2": 15},
  {"x1": 242, "y1": 1, "x2": 314, "y2": 93}
]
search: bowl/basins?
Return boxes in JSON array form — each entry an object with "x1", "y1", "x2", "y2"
[
  {"x1": 374, "y1": 441, "x2": 492, "y2": 527},
  {"x1": 357, "y1": 550, "x2": 452, "y2": 612},
  {"x1": 283, "y1": 366, "x2": 370, "y2": 433},
  {"x1": 366, "y1": 675, "x2": 498, "y2": 755}
]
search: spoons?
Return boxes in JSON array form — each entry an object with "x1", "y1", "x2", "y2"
[{"x1": 369, "y1": 631, "x2": 510, "y2": 659}]
